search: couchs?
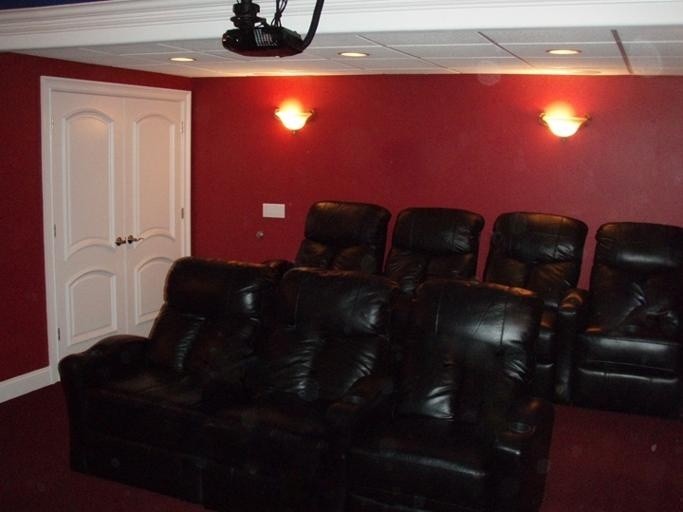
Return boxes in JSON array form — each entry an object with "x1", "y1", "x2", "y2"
[
  {"x1": 337, "y1": 276, "x2": 560, "y2": 511},
  {"x1": 203, "y1": 260, "x2": 402, "y2": 512},
  {"x1": 483, "y1": 212, "x2": 589, "y2": 406},
  {"x1": 383, "y1": 206, "x2": 485, "y2": 289},
  {"x1": 260, "y1": 199, "x2": 392, "y2": 276},
  {"x1": 58, "y1": 255, "x2": 290, "y2": 506},
  {"x1": 572, "y1": 220, "x2": 683, "y2": 420}
]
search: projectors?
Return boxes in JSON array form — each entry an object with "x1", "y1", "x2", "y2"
[{"x1": 219, "y1": 0, "x2": 324, "y2": 56}]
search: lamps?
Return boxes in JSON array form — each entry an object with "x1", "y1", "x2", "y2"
[
  {"x1": 538, "y1": 114, "x2": 590, "y2": 140},
  {"x1": 276, "y1": 109, "x2": 311, "y2": 136}
]
